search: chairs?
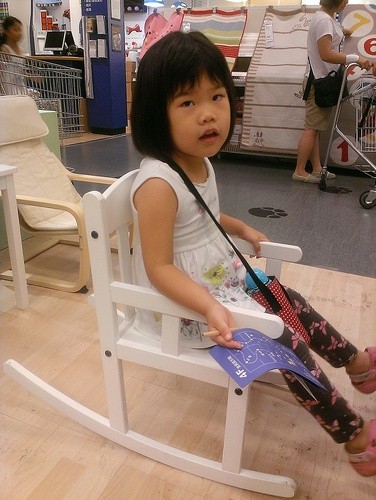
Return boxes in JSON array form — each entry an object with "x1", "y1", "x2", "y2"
[
  {"x1": 0, "y1": 93, "x2": 133, "y2": 293},
  {"x1": 1, "y1": 170, "x2": 304, "y2": 498}
]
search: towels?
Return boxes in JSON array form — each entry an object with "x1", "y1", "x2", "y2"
[
  {"x1": 140, "y1": 7, "x2": 248, "y2": 74},
  {"x1": 238, "y1": 7, "x2": 376, "y2": 174}
]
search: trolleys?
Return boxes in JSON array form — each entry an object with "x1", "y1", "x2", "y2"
[
  {"x1": 0, "y1": 51, "x2": 84, "y2": 184},
  {"x1": 318, "y1": 62, "x2": 376, "y2": 209}
]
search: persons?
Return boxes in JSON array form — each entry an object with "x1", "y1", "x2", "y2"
[
  {"x1": 0, "y1": 16, "x2": 40, "y2": 99},
  {"x1": 129, "y1": 30, "x2": 376, "y2": 477},
  {"x1": 291, "y1": 0, "x2": 372, "y2": 184}
]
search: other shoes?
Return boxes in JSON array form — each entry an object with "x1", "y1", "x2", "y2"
[
  {"x1": 291, "y1": 171, "x2": 321, "y2": 184},
  {"x1": 312, "y1": 170, "x2": 337, "y2": 180}
]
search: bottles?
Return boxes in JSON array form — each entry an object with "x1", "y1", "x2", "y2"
[{"x1": 245, "y1": 268, "x2": 273, "y2": 295}]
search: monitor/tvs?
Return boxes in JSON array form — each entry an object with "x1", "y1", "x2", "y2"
[{"x1": 43, "y1": 30, "x2": 66, "y2": 56}]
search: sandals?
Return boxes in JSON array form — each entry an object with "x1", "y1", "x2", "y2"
[
  {"x1": 350, "y1": 345, "x2": 376, "y2": 394},
  {"x1": 346, "y1": 418, "x2": 376, "y2": 477}
]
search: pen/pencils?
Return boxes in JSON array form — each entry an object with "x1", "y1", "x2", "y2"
[{"x1": 202, "y1": 327, "x2": 243, "y2": 337}]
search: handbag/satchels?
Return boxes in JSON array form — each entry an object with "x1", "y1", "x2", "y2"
[{"x1": 310, "y1": 64, "x2": 349, "y2": 107}]
